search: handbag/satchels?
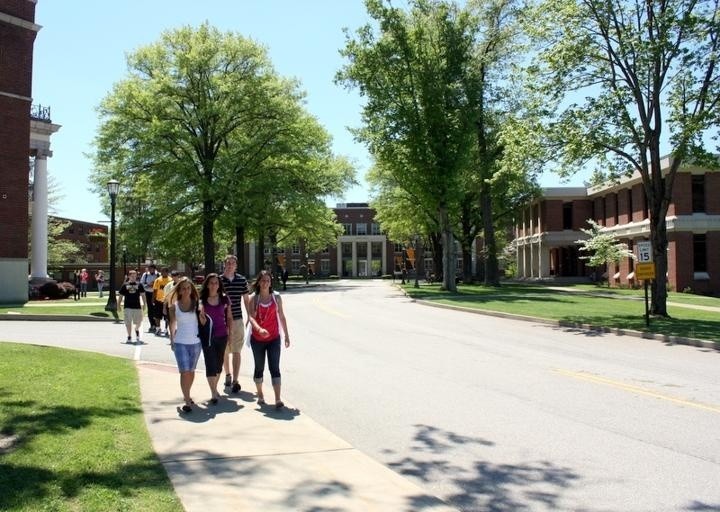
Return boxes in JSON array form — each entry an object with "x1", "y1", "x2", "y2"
[{"x1": 197, "y1": 311, "x2": 213, "y2": 349}]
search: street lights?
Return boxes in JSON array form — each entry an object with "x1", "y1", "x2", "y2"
[
  {"x1": 304, "y1": 250, "x2": 310, "y2": 286},
  {"x1": 400, "y1": 244, "x2": 407, "y2": 284},
  {"x1": 120, "y1": 244, "x2": 127, "y2": 280},
  {"x1": 100, "y1": 178, "x2": 124, "y2": 313},
  {"x1": 413, "y1": 231, "x2": 419, "y2": 289}
]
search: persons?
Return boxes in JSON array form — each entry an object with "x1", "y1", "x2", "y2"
[
  {"x1": 73, "y1": 269, "x2": 81, "y2": 300},
  {"x1": 95, "y1": 270, "x2": 106, "y2": 298},
  {"x1": 80, "y1": 269, "x2": 89, "y2": 297},
  {"x1": 117, "y1": 254, "x2": 290, "y2": 412}
]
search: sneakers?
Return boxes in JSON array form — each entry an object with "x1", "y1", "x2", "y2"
[
  {"x1": 126, "y1": 336, "x2": 132, "y2": 344},
  {"x1": 257, "y1": 399, "x2": 269, "y2": 410},
  {"x1": 149, "y1": 325, "x2": 169, "y2": 336},
  {"x1": 226, "y1": 374, "x2": 231, "y2": 386},
  {"x1": 182, "y1": 396, "x2": 195, "y2": 413},
  {"x1": 135, "y1": 330, "x2": 140, "y2": 338},
  {"x1": 232, "y1": 384, "x2": 241, "y2": 394},
  {"x1": 212, "y1": 393, "x2": 221, "y2": 406},
  {"x1": 276, "y1": 400, "x2": 283, "y2": 412}
]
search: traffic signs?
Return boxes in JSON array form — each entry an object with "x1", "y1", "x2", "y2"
[{"x1": 636, "y1": 240, "x2": 654, "y2": 263}]
